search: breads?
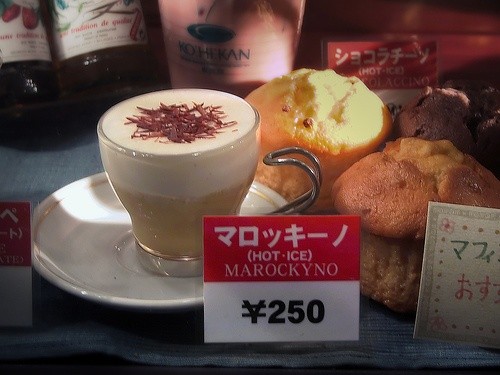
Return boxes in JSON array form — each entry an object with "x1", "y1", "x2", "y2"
[{"x1": 243, "y1": 66, "x2": 500, "y2": 313}]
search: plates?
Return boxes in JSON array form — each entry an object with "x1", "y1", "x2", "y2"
[{"x1": 31, "y1": 172, "x2": 301, "y2": 312}]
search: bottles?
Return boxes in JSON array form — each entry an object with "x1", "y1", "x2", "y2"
[
  {"x1": 47, "y1": 0, "x2": 171, "y2": 116},
  {"x1": 0, "y1": 0, "x2": 63, "y2": 151}
]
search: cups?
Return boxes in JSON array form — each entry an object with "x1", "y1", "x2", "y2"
[
  {"x1": 97, "y1": 88, "x2": 322, "y2": 261},
  {"x1": 158, "y1": 0, "x2": 307, "y2": 99}
]
